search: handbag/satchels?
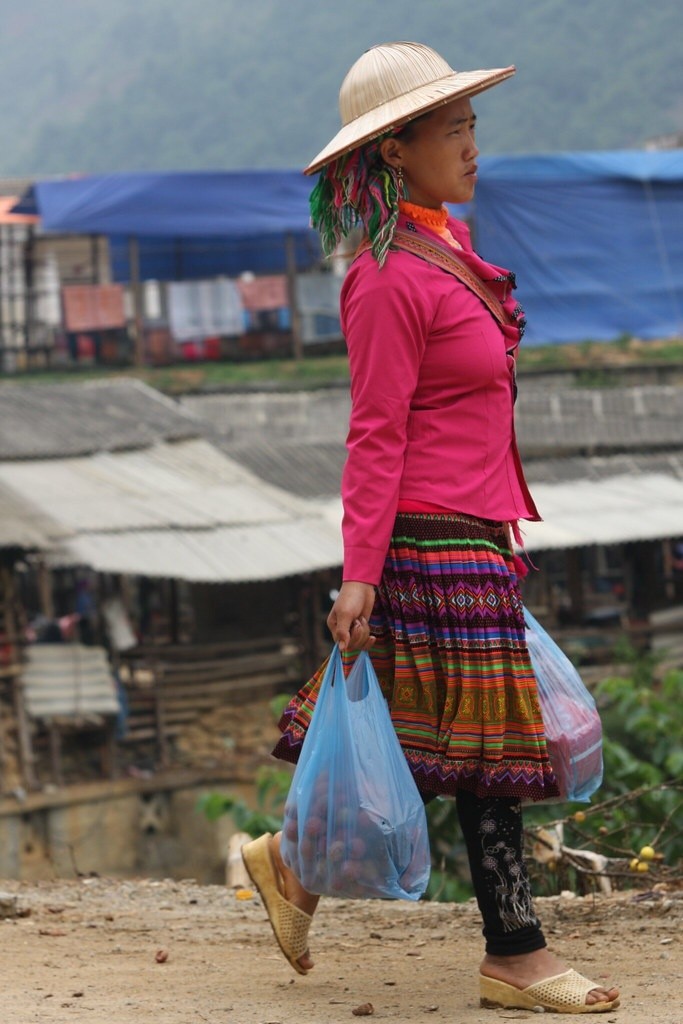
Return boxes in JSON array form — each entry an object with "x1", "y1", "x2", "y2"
[
  {"x1": 282, "y1": 629, "x2": 430, "y2": 902},
  {"x1": 519, "y1": 600, "x2": 604, "y2": 808}
]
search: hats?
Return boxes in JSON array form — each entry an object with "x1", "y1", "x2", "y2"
[{"x1": 303, "y1": 39, "x2": 512, "y2": 177}]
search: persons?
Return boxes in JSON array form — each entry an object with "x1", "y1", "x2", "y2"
[{"x1": 241, "y1": 45, "x2": 621, "y2": 1012}]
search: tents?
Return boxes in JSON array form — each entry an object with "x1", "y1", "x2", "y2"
[{"x1": 10, "y1": 147, "x2": 683, "y2": 358}]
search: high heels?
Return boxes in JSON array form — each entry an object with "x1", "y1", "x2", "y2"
[
  {"x1": 240, "y1": 830, "x2": 319, "y2": 974},
  {"x1": 477, "y1": 967, "x2": 620, "y2": 1013}
]
